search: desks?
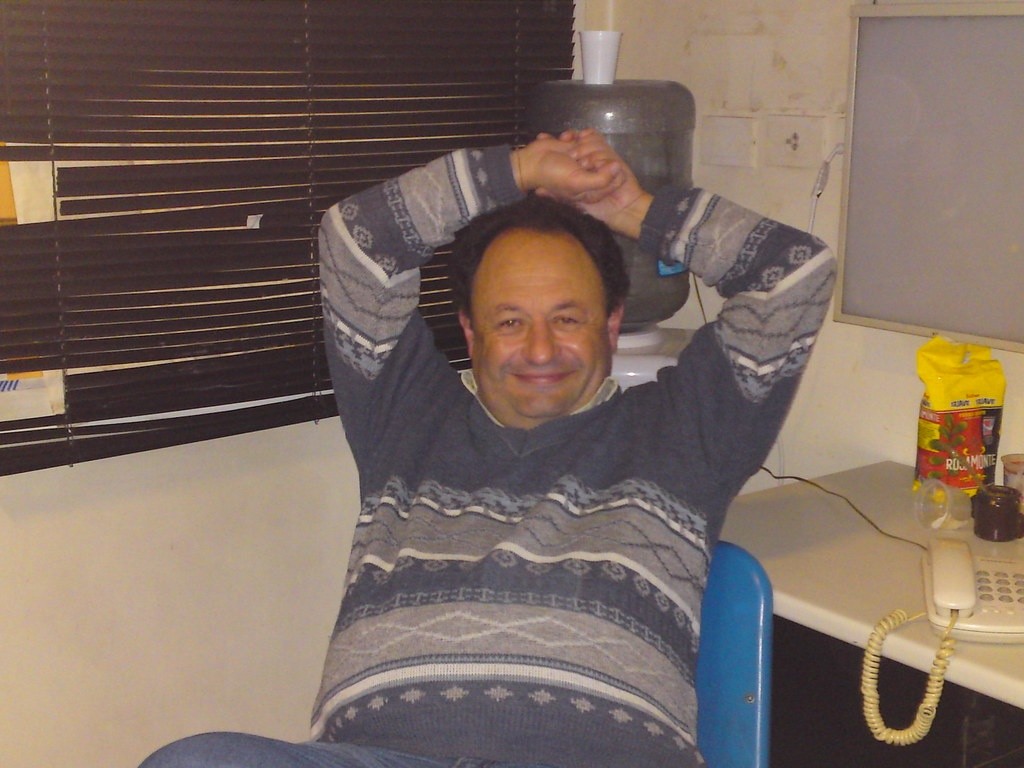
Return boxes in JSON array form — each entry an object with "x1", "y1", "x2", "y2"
[{"x1": 721, "y1": 461, "x2": 1024, "y2": 768}]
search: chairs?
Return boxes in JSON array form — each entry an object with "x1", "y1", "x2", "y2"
[{"x1": 697, "y1": 537, "x2": 773, "y2": 768}]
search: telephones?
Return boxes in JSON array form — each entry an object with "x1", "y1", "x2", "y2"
[{"x1": 922, "y1": 538, "x2": 1024, "y2": 644}]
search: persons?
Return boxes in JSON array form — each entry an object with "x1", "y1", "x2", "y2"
[{"x1": 138, "y1": 129, "x2": 838, "y2": 768}]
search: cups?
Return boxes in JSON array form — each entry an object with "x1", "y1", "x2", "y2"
[
  {"x1": 1001, "y1": 453, "x2": 1024, "y2": 538},
  {"x1": 974, "y1": 486, "x2": 1019, "y2": 542},
  {"x1": 578, "y1": 29, "x2": 623, "y2": 84},
  {"x1": 914, "y1": 478, "x2": 972, "y2": 532}
]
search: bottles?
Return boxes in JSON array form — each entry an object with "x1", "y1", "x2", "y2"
[{"x1": 525, "y1": 81, "x2": 697, "y2": 332}]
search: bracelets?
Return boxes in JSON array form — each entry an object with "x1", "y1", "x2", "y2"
[{"x1": 516, "y1": 147, "x2": 524, "y2": 191}]
